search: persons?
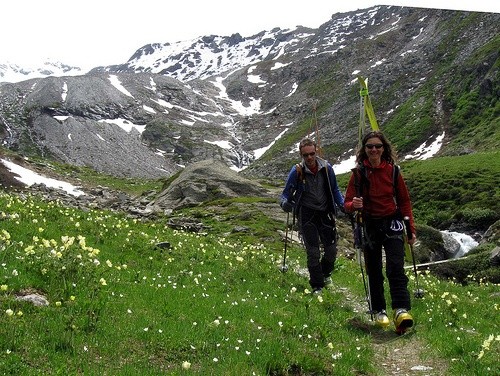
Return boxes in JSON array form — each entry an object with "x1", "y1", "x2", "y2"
[
  {"x1": 280, "y1": 139, "x2": 344, "y2": 294},
  {"x1": 344, "y1": 131, "x2": 417, "y2": 332}
]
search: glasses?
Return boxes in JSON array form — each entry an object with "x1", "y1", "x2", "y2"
[
  {"x1": 365, "y1": 144, "x2": 384, "y2": 149},
  {"x1": 302, "y1": 151, "x2": 315, "y2": 157}
]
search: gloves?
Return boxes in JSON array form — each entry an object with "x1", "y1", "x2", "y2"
[
  {"x1": 281, "y1": 201, "x2": 293, "y2": 212},
  {"x1": 339, "y1": 205, "x2": 345, "y2": 213}
]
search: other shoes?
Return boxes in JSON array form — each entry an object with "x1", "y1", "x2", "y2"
[
  {"x1": 393, "y1": 309, "x2": 413, "y2": 328},
  {"x1": 322, "y1": 275, "x2": 332, "y2": 288},
  {"x1": 314, "y1": 290, "x2": 322, "y2": 298},
  {"x1": 375, "y1": 310, "x2": 389, "y2": 325}
]
